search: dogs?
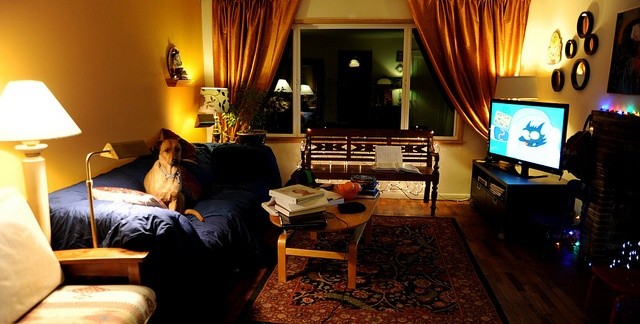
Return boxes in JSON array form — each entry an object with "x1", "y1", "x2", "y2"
[{"x1": 143, "y1": 138, "x2": 209, "y2": 223}]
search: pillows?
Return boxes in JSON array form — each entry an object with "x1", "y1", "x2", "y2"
[
  {"x1": 91, "y1": 187, "x2": 168, "y2": 209},
  {"x1": 160, "y1": 127, "x2": 213, "y2": 202}
]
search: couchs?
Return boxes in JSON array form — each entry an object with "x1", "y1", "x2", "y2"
[{"x1": 48, "y1": 143, "x2": 283, "y2": 306}]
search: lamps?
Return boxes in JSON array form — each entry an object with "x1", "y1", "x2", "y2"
[
  {"x1": 375, "y1": 76, "x2": 393, "y2": 107},
  {"x1": 85, "y1": 140, "x2": 152, "y2": 249},
  {"x1": 494, "y1": 76, "x2": 539, "y2": 103},
  {"x1": 0, "y1": 81, "x2": 83, "y2": 247},
  {"x1": 199, "y1": 86, "x2": 232, "y2": 142},
  {"x1": 273, "y1": 79, "x2": 293, "y2": 103},
  {"x1": 301, "y1": 85, "x2": 315, "y2": 110},
  {"x1": 348, "y1": 58, "x2": 360, "y2": 68}
]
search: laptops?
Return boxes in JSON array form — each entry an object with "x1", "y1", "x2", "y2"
[{"x1": 280, "y1": 212, "x2": 327, "y2": 229}]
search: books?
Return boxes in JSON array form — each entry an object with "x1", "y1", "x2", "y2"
[
  {"x1": 489, "y1": 183, "x2": 505, "y2": 197},
  {"x1": 259, "y1": 182, "x2": 345, "y2": 230},
  {"x1": 350, "y1": 174, "x2": 379, "y2": 200}
]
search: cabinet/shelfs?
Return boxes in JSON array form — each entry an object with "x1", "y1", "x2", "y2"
[{"x1": 470, "y1": 158, "x2": 571, "y2": 245}]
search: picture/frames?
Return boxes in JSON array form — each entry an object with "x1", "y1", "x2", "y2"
[
  {"x1": 565, "y1": 40, "x2": 578, "y2": 58},
  {"x1": 584, "y1": 34, "x2": 598, "y2": 55},
  {"x1": 551, "y1": 69, "x2": 563, "y2": 92},
  {"x1": 577, "y1": 11, "x2": 594, "y2": 38},
  {"x1": 571, "y1": 59, "x2": 590, "y2": 90}
]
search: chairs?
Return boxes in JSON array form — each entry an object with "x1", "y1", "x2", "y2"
[{"x1": 0, "y1": 185, "x2": 158, "y2": 324}]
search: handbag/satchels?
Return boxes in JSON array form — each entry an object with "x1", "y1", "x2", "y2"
[{"x1": 559, "y1": 114, "x2": 598, "y2": 183}]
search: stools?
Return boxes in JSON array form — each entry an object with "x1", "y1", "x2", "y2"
[{"x1": 582, "y1": 263, "x2": 639, "y2": 324}]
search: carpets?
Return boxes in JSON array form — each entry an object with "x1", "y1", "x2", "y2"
[{"x1": 233, "y1": 216, "x2": 511, "y2": 324}]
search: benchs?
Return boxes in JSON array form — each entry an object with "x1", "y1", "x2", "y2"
[{"x1": 299, "y1": 128, "x2": 440, "y2": 216}]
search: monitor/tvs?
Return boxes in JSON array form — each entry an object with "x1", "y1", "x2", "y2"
[{"x1": 488, "y1": 98, "x2": 569, "y2": 179}]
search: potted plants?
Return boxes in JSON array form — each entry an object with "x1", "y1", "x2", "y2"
[{"x1": 222, "y1": 82, "x2": 287, "y2": 144}]
